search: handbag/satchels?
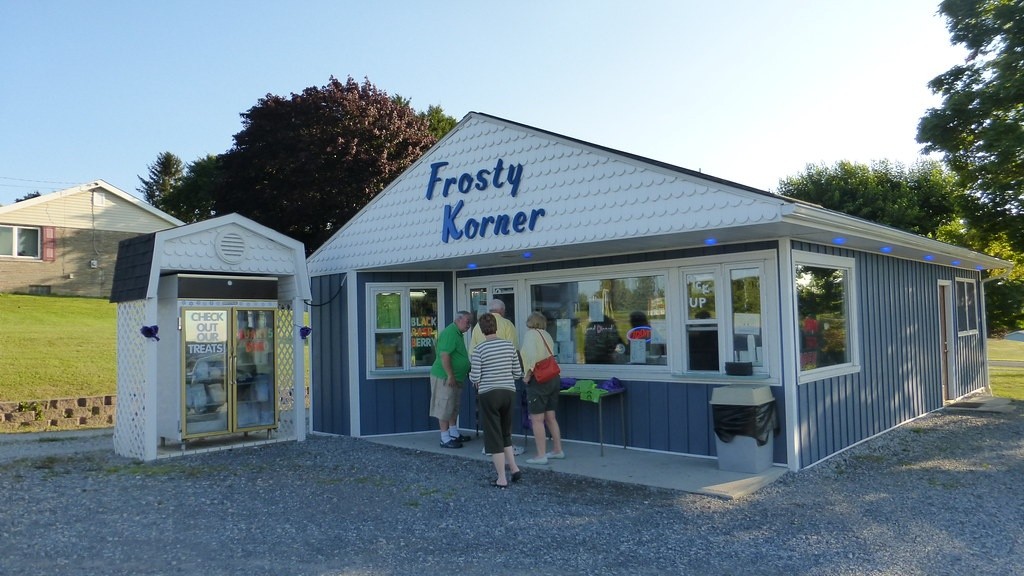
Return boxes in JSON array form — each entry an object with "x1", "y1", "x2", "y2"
[{"x1": 533, "y1": 354, "x2": 561, "y2": 384}]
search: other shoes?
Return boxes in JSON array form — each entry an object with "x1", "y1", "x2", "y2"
[
  {"x1": 525, "y1": 457, "x2": 548, "y2": 465},
  {"x1": 512, "y1": 444, "x2": 527, "y2": 456},
  {"x1": 440, "y1": 439, "x2": 463, "y2": 448},
  {"x1": 450, "y1": 434, "x2": 471, "y2": 442},
  {"x1": 546, "y1": 450, "x2": 565, "y2": 459}
]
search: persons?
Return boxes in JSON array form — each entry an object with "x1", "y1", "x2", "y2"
[
  {"x1": 628, "y1": 311, "x2": 667, "y2": 366},
  {"x1": 469, "y1": 311, "x2": 522, "y2": 490},
  {"x1": 429, "y1": 311, "x2": 472, "y2": 449},
  {"x1": 520, "y1": 311, "x2": 567, "y2": 465},
  {"x1": 468, "y1": 299, "x2": 525, "y2": 457},
  {"x1": 584, "y1": 300, "x2": 628, "y2": 364}
]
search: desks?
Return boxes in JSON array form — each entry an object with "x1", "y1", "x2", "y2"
[{"x1": 549, "y1": 386, "x2": 627, "y2": 458}]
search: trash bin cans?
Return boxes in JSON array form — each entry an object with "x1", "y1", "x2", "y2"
[{"x1": 708, "y1": 385, "x2": 776, "y2": 474}]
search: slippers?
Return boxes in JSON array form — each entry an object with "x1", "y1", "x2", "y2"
[
  {"x1": 509, "y1": 471, "x2": 522, "y2": 482},
  {"x1": 490, "y1": 479, "x2": 507, "y2": 489}
]
